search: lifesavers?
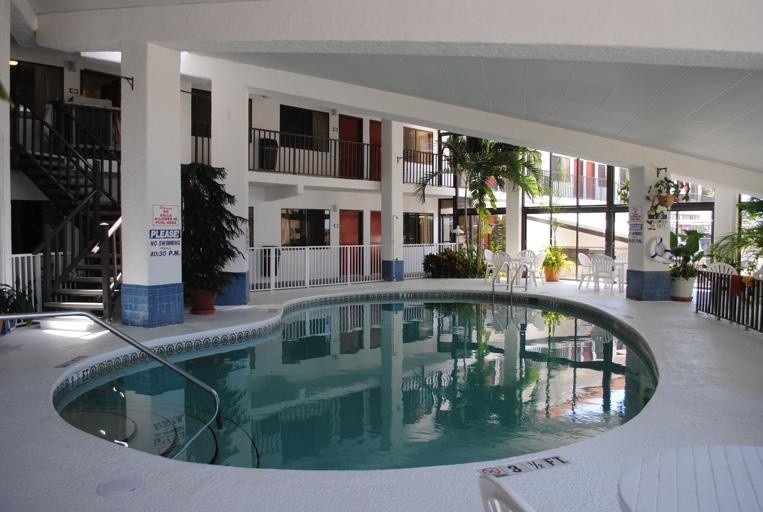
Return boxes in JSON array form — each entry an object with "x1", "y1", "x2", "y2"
[{"x1": 649, "y1": 236, "x2": 671, "y2": 263}]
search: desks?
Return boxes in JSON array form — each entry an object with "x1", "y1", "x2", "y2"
[
  {"x1": 599, "y1": 258, "x2": 632, "y2": 290},
  {"x1": 500, "y1": 256, "x2": 539, "y2": 277}
]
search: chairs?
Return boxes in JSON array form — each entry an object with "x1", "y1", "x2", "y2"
[
  {"x1": 591, "y1": 254, "x2": 618, "y2": 291},
  {"x1": 707, "y1": 261, "x2": 738, "y2": 277},
  {"x1": 524, "y1": 254, "x2": 544, "y2": 287},
  {"x1": 484, "y1": 250, "x2": 498, "y2": 282},
  {"x1": 579, "y1": 254, "x2": 608, "y2": 290},
  {"x1": 518, "y1": 250, "x2": 538, "y2": 283},
  {"x1": 492, "y1": 250, "x2": 520, "y2": 285}
]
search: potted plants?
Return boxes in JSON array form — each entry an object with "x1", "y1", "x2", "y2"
[
  {"x1": 180, "y1": 161, "x2": 251, "y2": 314},
  {"x1": 542, "y1": 244, "x2": 567, "y2": 282},
  {"x1": 644, "y1": 174, "x2": 690, "y2": 224},
  {"x1": 663, "y1": 228, "x2": 709, "y2": 300}
]
